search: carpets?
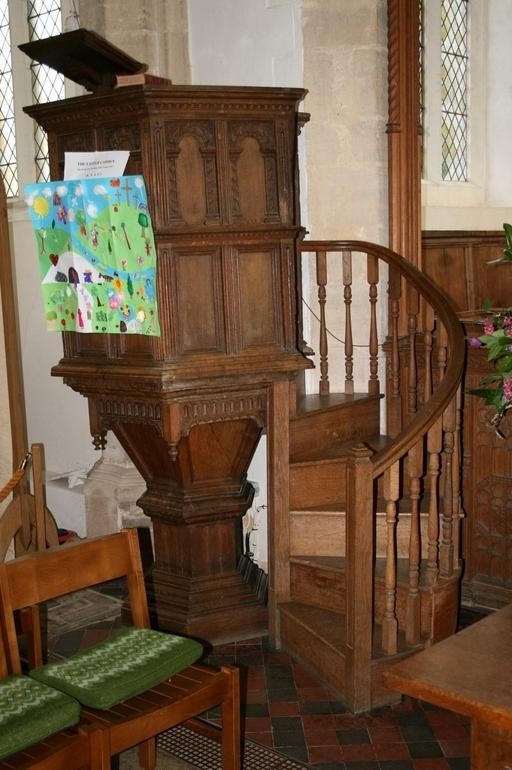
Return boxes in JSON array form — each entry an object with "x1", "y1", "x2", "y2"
[{"x1": 85, "y1": 715, "x2": 319, "y2": 770}]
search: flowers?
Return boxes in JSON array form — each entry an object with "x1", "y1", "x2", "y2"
[{"x1": 462, "y1": 221, "x2": 511, "y2": 443}]
